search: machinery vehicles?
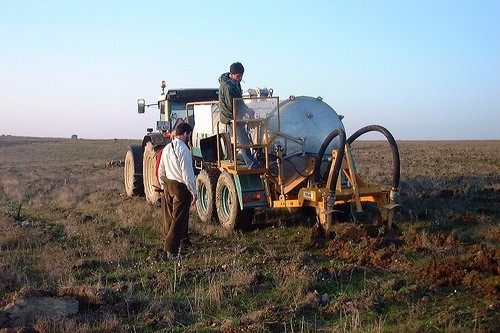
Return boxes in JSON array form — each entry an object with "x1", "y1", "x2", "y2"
[{"x1": 123, "y1": 80, "x2": 403, "y2": 232}]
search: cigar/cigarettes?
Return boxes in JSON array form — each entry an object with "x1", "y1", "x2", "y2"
[{"x1": 241, "y1": 80, "x2": 245, "y2": 83}]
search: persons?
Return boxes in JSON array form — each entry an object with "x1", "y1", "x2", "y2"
[
  {"x1": 153, "y1": 122, "x2": 197, "y2": 258},
  {"x1": 218, "y1": 62, "x2": 264, "y2": 169}
]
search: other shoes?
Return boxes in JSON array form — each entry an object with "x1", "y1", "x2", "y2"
[
  {"x1": 167, "y1": 247, "x2": 191, "y2": 260},
  {"x1": 181, "y1": 243, "x2": 201, "y2": 254},
  {"x1": 253, "y1": 162, "x2": 264, "y2": 169}
]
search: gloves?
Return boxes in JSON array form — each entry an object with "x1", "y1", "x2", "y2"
[
  {"x1": 246, "y1": 108, "x2": 255, "y2": 119},
  {"x1": 238, "y1": 108, "x2": 245, "y2": 118}
]
